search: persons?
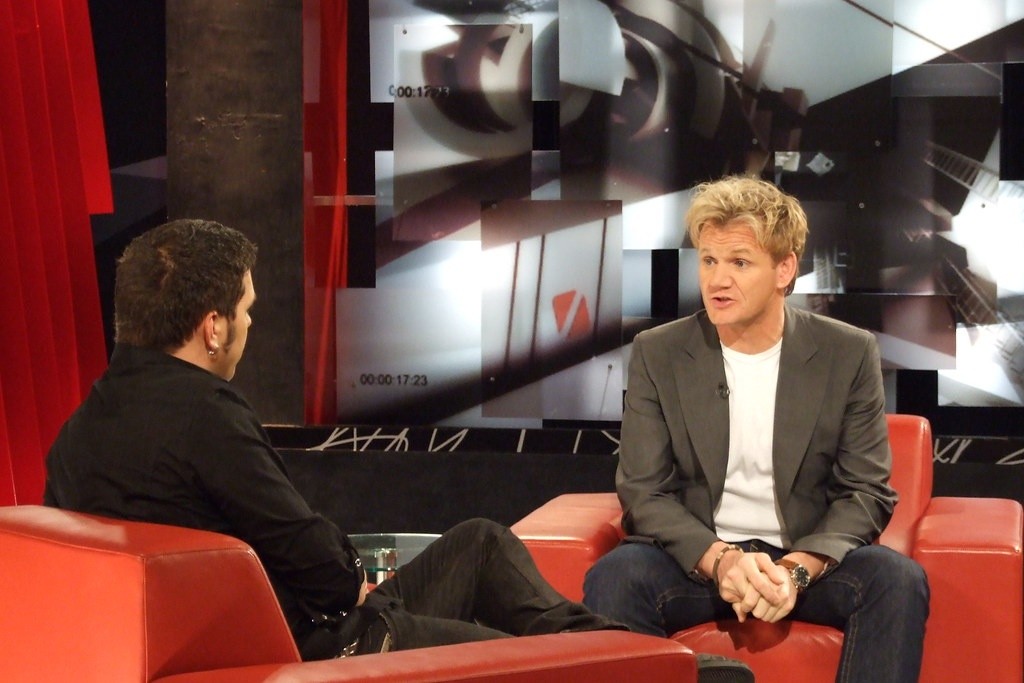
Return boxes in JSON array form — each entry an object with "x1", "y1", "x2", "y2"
[
  {"x1": 581, "y1": 178, "x2": 930, "y2": 683},
  {"x1": 45, "y1": 219, "x2": 755, "y2": 683}
]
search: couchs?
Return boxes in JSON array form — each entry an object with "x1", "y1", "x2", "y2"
[
  {"x1": 0, "y1": 504, "x2": 699, "y2": 683},
  {"x1": 508, "y1": 414, "x2": 1024, "y2": 682}
]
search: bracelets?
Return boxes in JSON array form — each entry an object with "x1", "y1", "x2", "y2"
[{"x1": 713, "y1": 545, "x2": 744, "y2": 587}]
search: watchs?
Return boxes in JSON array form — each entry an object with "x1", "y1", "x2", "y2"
[{"x1": 775, "y1": 558, "x2": 810, "y2": 592}]
